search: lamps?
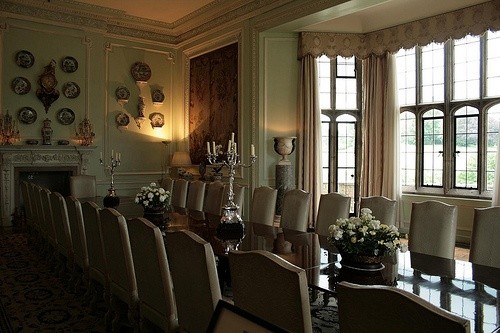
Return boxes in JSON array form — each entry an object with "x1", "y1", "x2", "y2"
[
  {"x1": 170, "y1": 151, "x2": 193, "y2": 180},
  {"x1": 0, "y1": 108, "x2": 20, "y2": 145},
  {"x1": 204, "y1": 130, "x2": 255, "y2": 231},
  {"x1": 74, "y1": 119, "x2": 95, "y2": 146},
  {"x1": 99, "y1": 148, "x2": 121, "y2": 197}
]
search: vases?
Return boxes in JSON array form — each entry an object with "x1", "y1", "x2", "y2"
[
  {"x1": 273, "y1": 136, "x2": 297, "y2": 165},
  {"x1": 41, "y1": 119, "x2": 53, "y2": 145},
  {"x1": 334, "y1": 242, "x2": 390, "y2": 271},
  {"x1": 142, "y1": 203, "x2": 165, "y2": 214}
]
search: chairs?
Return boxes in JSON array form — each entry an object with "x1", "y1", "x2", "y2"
[{"x1": 20, "y1": 175, "x2": 500, "y2": 333}]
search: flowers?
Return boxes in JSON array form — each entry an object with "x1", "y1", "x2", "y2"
[
  {"x1": 134, "y1": 182, "x2": 172, "y2": 211},
  {"x1": 327, "y1": 207, "x2": 408, "y2": 258}
]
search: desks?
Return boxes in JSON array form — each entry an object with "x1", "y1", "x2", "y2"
[{"x1": 71, "y1": 196, "x2": 500, "y2": 333}]
